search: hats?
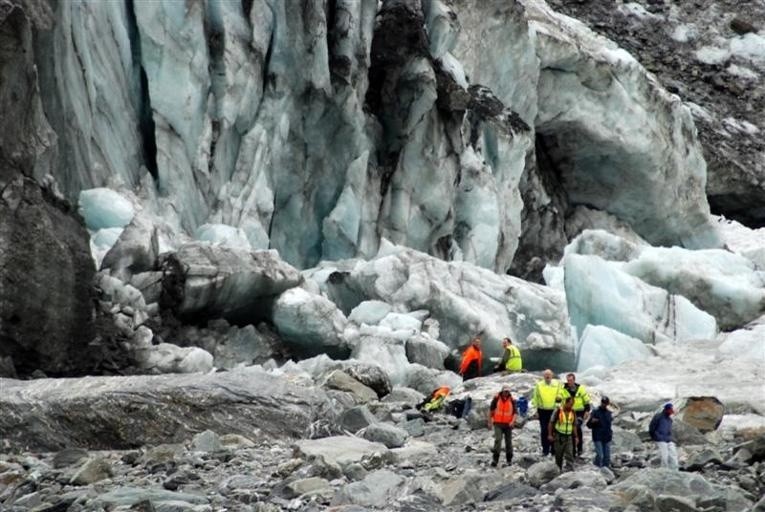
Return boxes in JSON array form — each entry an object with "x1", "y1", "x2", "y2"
[
  {"x1": 502, "y1": 383, "x2": 510, "y2": 391},
  {"x1": 663, "y1": 403, "x2": 676, "y2": 415}
]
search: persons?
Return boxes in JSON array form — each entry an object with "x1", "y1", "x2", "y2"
[
  {"x1": 647, "y1": 404, "x2": 679, "y2": 471},
  {"x1": 491, "y1": 340, "x2": 522, "y2": 373},
  {"x1": 462, "y1": 339, "x2": 482, "y2": 380},
  {"x1": 489, "y1": 386, "x2": 519, "y2": 467},
  {"x1": 533, "y1": 369, "x2": 614, "y2": 472}
]
